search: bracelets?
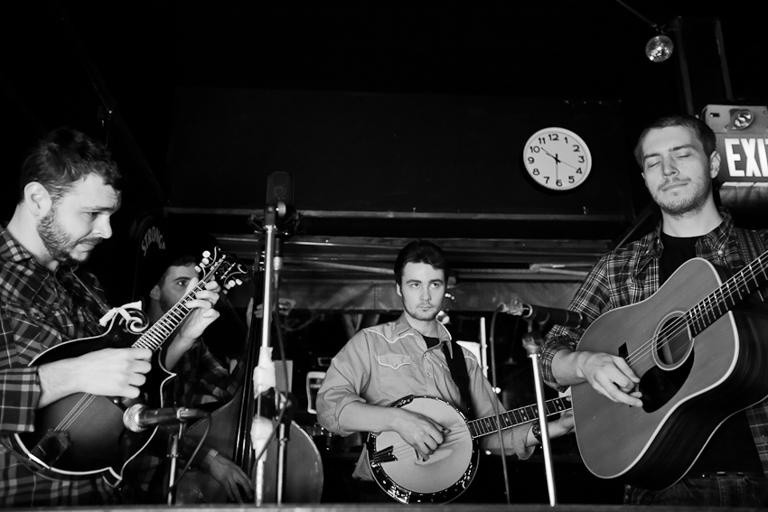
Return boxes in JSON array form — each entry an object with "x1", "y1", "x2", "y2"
[{"x1": 532, "y1": 423, "x2": 542, "y2": 442}]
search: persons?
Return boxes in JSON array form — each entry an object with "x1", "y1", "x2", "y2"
[
  {"x1": 138, "y1": 219, "x2": 276, "y2": 504},
  {"x1": 538, "y1": 114, "x2": 768, "y2": 508},
  {"x1": 315, "y1": 240, "x2": 575, "y2": 504},
  {"x1": 0, "y1": 128, "x2": 220, "y2": 509}
]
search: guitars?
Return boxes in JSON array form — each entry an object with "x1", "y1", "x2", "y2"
[
  {"x1": 571, "y1": 250, "x2": 768, "y2": 494},
  {"x1": 366, "y1": 394, "x2": 573, "y2": 504},
  {"x1": 9, "y1": 244, "x2": 250, "y2": 488}
]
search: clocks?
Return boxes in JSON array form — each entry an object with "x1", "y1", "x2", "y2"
[{"x1": 520, "y1": 123, "x2": 595, "y2": 196}]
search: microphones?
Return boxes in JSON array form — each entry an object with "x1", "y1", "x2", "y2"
[
  {"x1": 256, "y1": 201, "x2": 286, "y2": 217},
  {"x1": 500, "y1": 303, "x2": 588, "y2": 328},
  {"x1": 272, "y1": 234, "x2": 286, "y2": 272},
  {"x1": 122, "y1": 403, "x2": 198, "y2": 432}
]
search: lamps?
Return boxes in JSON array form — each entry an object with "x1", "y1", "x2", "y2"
[{"x1": 640, "y1": 29, "x2": 675, "y2": 65}]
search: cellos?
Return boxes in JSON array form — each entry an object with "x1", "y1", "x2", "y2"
[{"x1": 174, "y1": 210, "x2": 326, "y2": 502}]
